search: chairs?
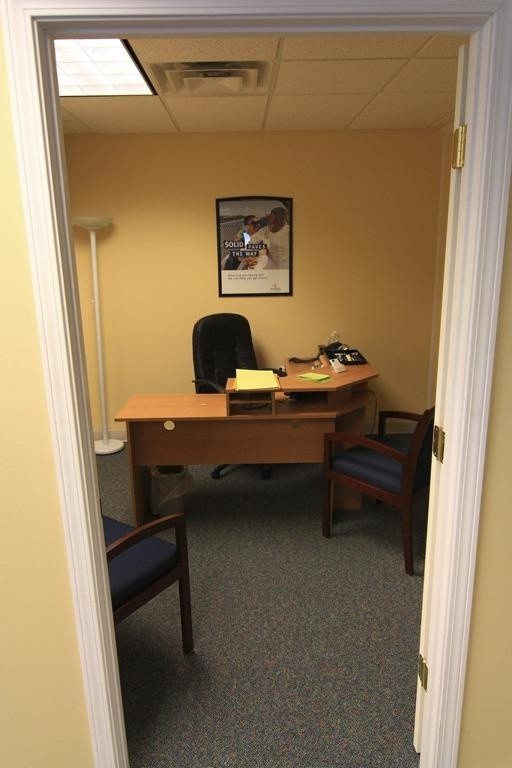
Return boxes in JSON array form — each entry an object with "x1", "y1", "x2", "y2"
[
  {"x1": 322, "y1": 405, "x2": 435, "y2": 575},
  {"x1": 192, "y1": 313, "x2": 287, "y2": 479},
  {"x1": 100, "y1": 498, "x2": 194, "y2": 654}
]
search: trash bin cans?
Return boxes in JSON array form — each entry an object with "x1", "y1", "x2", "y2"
[{"x1": 150, "y1": 465, "x2": 187, "y2": 517}]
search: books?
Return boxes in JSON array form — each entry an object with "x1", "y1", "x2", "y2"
[{"x1": 230, "y1": 369, "x2": 281, "y2": 414}]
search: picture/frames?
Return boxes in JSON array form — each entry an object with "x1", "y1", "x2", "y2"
[{"x1": 215, "y1": 196, "x2": 292, "y2": 297}]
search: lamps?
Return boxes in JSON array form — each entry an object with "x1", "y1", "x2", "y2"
[{"x1": 67, "y1": 217, "x2": 125, "y2": 455}]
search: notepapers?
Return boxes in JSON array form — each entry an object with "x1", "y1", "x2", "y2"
[{"x1": 298, "y1": 372, "x2": 329, "y2": 381}]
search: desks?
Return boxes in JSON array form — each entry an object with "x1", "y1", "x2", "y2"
[{"x1": 114, "y1": 358, "x2": 381, "y2": 530}]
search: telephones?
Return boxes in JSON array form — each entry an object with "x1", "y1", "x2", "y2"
[{"x1": 317, "y1": 341, "x2": 342, "y2": 358}]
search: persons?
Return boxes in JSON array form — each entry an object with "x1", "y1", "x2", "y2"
[
  {"x1": 222, "y1": 214, "x2": 268, "y2": 271},
  {"x1": 245, "y1": 206, "x2": 290, "y2": 270}
]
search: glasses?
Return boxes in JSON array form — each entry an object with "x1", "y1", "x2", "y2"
[{"x1": 247, "y1": 220, "x2": 259, "y2": 226}]
